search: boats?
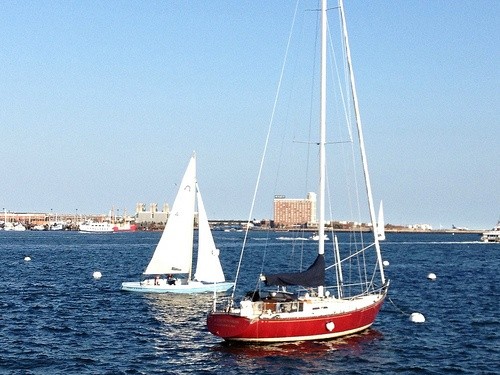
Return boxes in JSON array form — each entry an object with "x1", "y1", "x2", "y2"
[
  {"x1": 308, "y1": 231, "x2": 330, "y2": 241},
  {"x1": 481, "y1": 221, "x2": 500, "y2": 243},
  {"x1": 1, "y1": 205, "x2": 138, "y2": 233}
]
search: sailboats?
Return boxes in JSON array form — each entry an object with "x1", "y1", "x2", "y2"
[
  {"x1": 375, "y1": 200, "x2": 385, "y2": 241},
  {"x1": 204, "y1": 0, "x2": 391, "y2": 346},
  {"x1": 118, "y1": 147, "x2": 236, "y2": 295}
]
